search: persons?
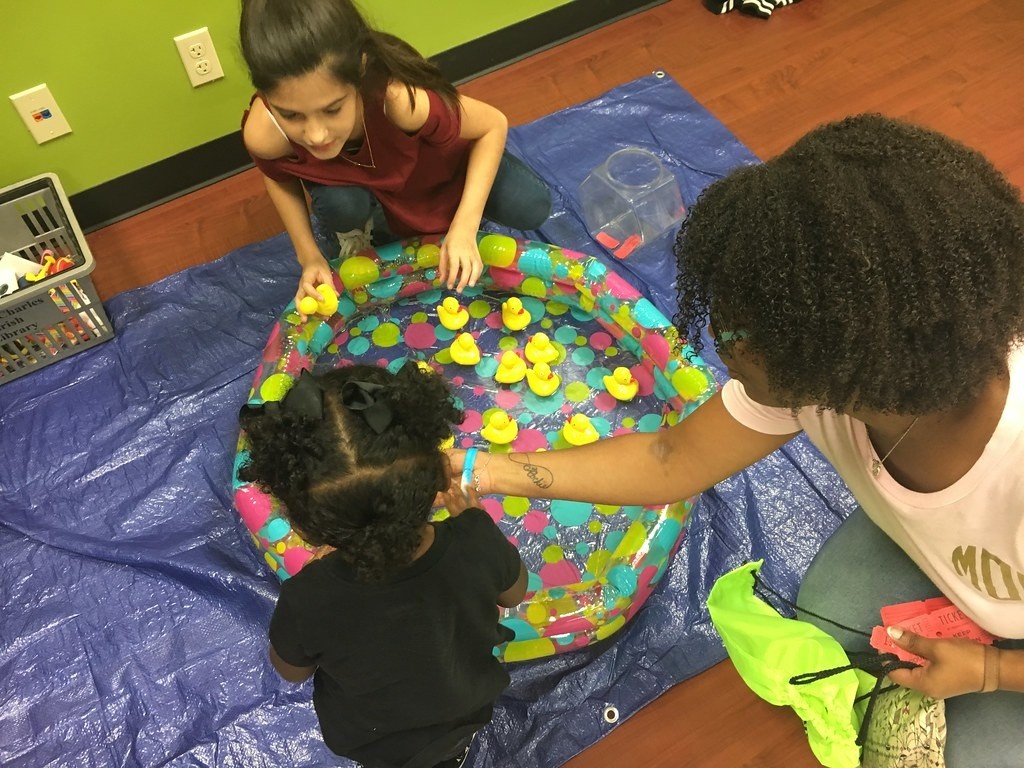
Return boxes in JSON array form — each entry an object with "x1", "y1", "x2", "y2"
[
  {"x1": 240, "y1": 1, "x2": 552, "y2": 326},
  {"x1": 237, "y1": 360, "x2": 529, "y2": 768},
  {"x1": 437, "y1": 111, "x2": 1024, "y2": 768}
]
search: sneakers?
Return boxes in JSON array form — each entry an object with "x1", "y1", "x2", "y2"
[{"x1": 336, "y1": 214, "x2": 378, "y2": 259}]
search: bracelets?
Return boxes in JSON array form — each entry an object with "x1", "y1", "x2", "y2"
[
  {"x1": 473, "y1": 454, "x2": 494, "y2": 492},
  {"x1": 459, "y1": 447, "x2": 478, "y2": 497}
]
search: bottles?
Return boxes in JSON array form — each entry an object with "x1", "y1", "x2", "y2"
[{"x1": 577, "y1": 148, "x2": 686, "y2": 259}]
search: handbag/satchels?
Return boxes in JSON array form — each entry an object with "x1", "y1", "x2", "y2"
[{"x1": 706, "y1": 554, "x2": 924, "y2": 767}]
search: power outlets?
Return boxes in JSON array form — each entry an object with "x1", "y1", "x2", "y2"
[
  {"x1": 174, "y1": 27, "x2": 224, "y2": 88},
  {"x1": 8, "y1": 82, "x2": 73, "y2": 145}
]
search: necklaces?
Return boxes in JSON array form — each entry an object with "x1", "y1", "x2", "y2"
[
  {"x1": 859, "y1": 416, "x2": 924, "y2": 479},
  {"x1": 336, "y1": 93, "x2": 376, "y2": 169}
]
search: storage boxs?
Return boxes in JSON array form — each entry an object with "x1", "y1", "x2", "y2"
[{"x1": 0, "y1": 172, "x2": 116, "y2": 389}]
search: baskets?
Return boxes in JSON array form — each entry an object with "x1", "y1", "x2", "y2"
[{"x1": 0, "y1": 173, "x2": 115, "y2": 390}]
